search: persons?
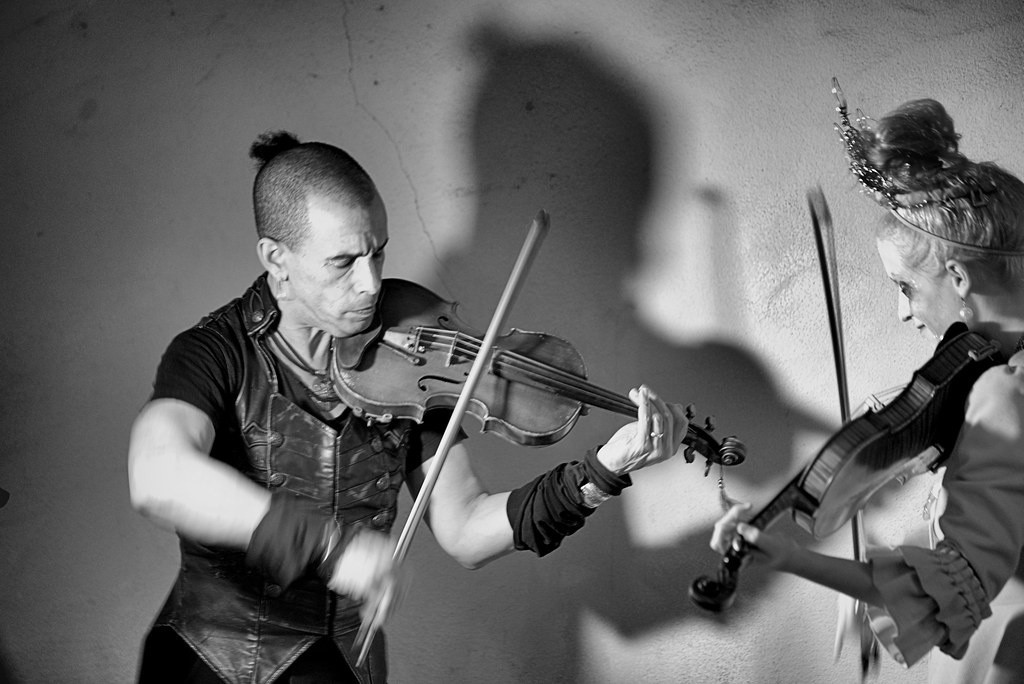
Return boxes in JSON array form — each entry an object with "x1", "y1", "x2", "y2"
[
  {"x1": 710, "y1": 79, "x2": 1024, "y2": 684},
  {"x1": 129, "y1": 131, "x2": 689, "y2": 684}
]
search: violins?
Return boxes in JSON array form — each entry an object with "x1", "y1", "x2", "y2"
[
  {"x1": 329, "y1": 278, "x2": 749, "y2": 479},
  {"x1": 686, "y1": 328, "x2": 1003, "y2": 616}
]
